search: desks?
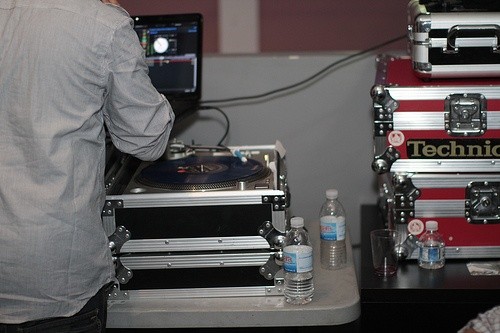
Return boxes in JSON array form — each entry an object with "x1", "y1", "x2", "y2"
[
  {"x1": 357, "y1": 202, "x2": 499, "y2": 333},
  {"x1": 106, "y1": 215, "x2": 358, "y2": 333}
]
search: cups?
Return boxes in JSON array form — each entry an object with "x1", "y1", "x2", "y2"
[{"x1": 370, "y1": 229, "x2": 401, "y2": 277}]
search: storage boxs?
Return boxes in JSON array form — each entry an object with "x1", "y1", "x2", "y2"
[
  {"x1": 370, "y1": 53, "x2": 500, "y2": 173},
  {"x1": 99, "y1": 142, "x2": 291, "y2": 304},
  {"x1": 378, "y1": 170, "x2": 500, "y2": 261},
  {"x1": 406, "y1": 0, "x2": 500, "y2": 80}
]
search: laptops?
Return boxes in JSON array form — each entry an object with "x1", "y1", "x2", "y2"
[{"x1": 131, "y1": 13, "x2": 203, "y2": 117}]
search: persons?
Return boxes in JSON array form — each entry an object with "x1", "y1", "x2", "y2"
[{"x1": 0, "y1": 0, "x2": 175, "y2": 333}]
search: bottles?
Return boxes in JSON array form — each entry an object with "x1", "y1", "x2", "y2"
[
  {"x1": 419, "y1": 221, "x2": 446, "y2": 271},
  {"x1": 320, "y1": 190, "x2": 347, "y2": 270},
  {"x1": 284, "y1": 217, "x2": 314, "y2": 305}
]
